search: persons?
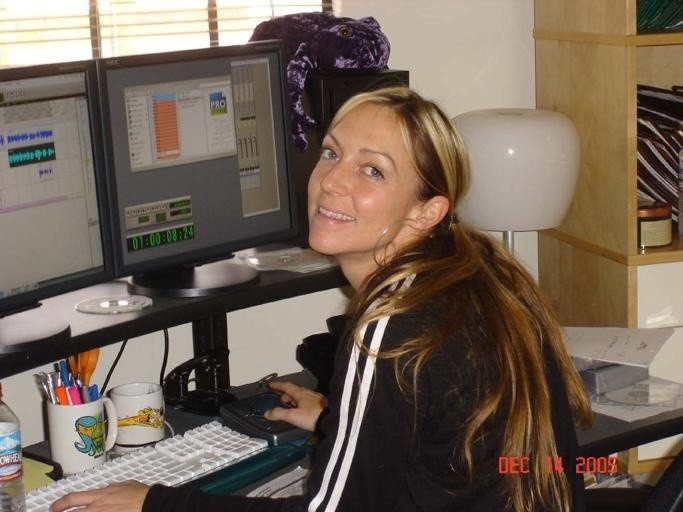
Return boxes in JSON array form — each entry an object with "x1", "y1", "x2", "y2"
[{"x1": 49, "y1": 85, "x2": 594, "y2": 512}]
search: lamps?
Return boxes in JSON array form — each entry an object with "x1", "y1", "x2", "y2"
[{"x1": 452, "y1": 106, "x2": 583, "y2": 277}]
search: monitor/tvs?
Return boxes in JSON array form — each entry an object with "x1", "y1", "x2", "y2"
[
  {"x1": 0, "y1": 59, "x2": 116, "y2": 353},
  {"x1": 95, "y1": 38, "x2": 300, "y2": 299}
]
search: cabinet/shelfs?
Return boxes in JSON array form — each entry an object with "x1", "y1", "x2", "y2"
[{"x1": 534, "y1": 1, "x2": 682, "y2": 330}]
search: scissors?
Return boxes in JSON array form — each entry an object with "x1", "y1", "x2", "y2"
[{"x1": 68, "y1": 348, "x2": 99, "y2": 387}]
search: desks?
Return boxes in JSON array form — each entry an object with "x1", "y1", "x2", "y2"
[{"x1": 244, "y1": 353, "x2": 683, "y2": 507}]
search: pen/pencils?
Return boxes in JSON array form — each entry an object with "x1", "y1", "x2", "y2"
[{"x1": 34, "y1": 360, "x2": 98, "y2": 406}]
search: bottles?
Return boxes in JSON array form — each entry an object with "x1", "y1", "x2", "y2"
[{"x1": 0, "y1": 383, "x2": 26, "y2": 512}]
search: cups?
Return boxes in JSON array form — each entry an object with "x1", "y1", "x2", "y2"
[{"x1": 47, "y1": 382, "x2": 165, "y2": 478}]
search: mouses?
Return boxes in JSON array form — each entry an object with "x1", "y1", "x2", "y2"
[{"x1": 250, "y1": 392, "x2": 296, "y2": 417}]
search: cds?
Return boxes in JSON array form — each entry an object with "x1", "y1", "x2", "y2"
[
  {"x1": 75, "y1": 295, "x2": 153, "y2": 314},
  {"x1": 246, "y1": 248, "x2": 329, "y2": 270},
  {"x1": 605, "y1": 383, "x2": 675, "y2": 406}
]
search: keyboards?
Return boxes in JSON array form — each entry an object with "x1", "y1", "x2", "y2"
[{"x1": 24, "y1": 419, "x2": 269, "y2": 512}]
